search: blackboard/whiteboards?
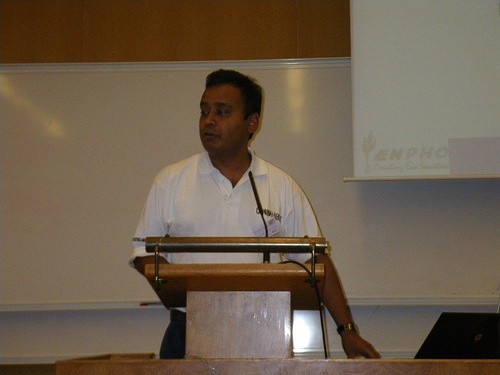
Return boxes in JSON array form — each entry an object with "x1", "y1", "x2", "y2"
[{"x1": 0, "y1": 55, "x2": 500, "y2": 314}]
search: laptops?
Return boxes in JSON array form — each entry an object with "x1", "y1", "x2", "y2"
[{"x1": 414, "y1": 312, "x2": 500, "y2": 360}]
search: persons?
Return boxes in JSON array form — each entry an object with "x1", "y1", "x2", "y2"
[{"x1": 127, "y1": 68, "x2": 383, "y2": 360}]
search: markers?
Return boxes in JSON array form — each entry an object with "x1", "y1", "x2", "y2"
[{"x1": 140, "y1": 301, "x2": 163, "y2": 307}]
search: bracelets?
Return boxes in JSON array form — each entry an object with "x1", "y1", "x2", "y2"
[{"x1": 336, "y1": 321, "x2": 360, "y2": 335}]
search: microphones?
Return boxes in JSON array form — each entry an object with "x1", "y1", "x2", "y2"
[{"x1": 249, "y1": 171, "x2": 270, "y2": 263}]
